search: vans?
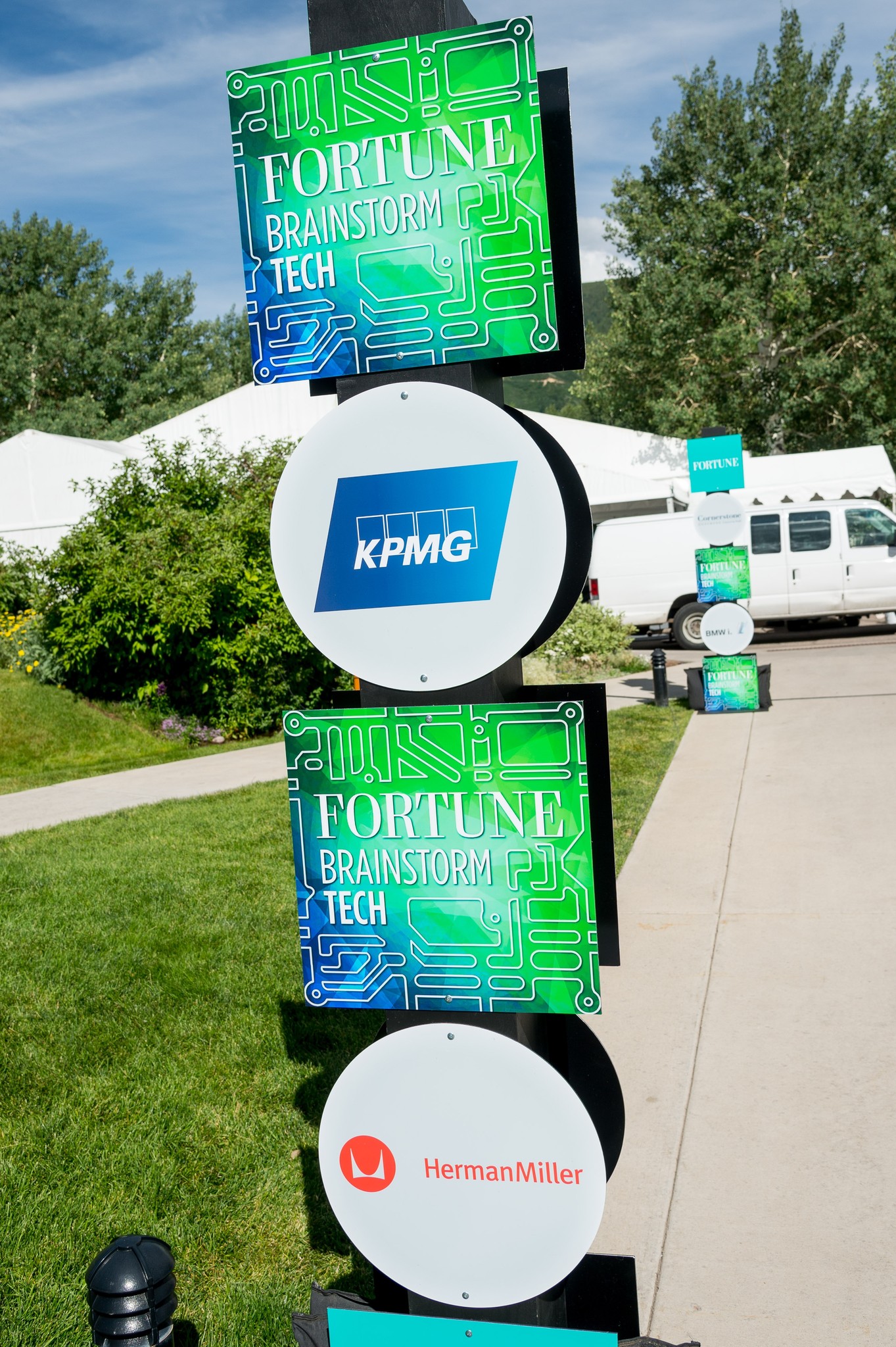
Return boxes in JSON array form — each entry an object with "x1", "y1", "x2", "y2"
[{"x1": 588, "y1": 499, "x2": 896, "y2": 650}]
